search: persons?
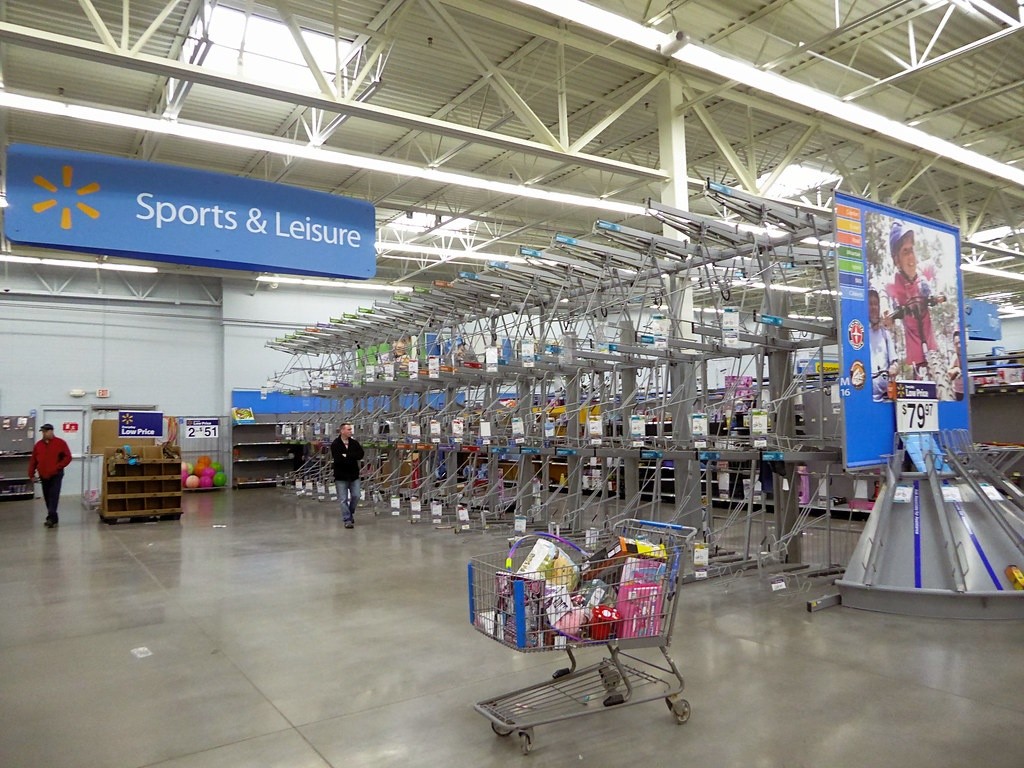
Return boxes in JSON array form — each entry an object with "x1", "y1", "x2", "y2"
[
  {"x1": 867, "y1": 286, "x2": 900, "y2": 401},
  {"x1": 28, "y1": 424, "x2": 73, "y2": 528},
  {"x1": 884, "y1": 221, "x2": 945, "y2": 365},
  {"x1": 330, "y1": 423, "x2": 365, "y2": 528},
  {"x1": 948, "y1": 331, "x2": 963, "y2": 401}
]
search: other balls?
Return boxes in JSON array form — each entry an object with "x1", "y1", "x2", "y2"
[{"x1": 180, "y1": 452, "x2": 226, "y2": 490}]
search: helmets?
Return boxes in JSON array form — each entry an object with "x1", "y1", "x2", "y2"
[{"x1": 890, "y1": 222, "x2": 914, "y2": 253}]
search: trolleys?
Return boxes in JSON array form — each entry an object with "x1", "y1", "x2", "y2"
[
  {"x1": 467, "y1": 517, "x2": 697, "y2": 757},
  {"x1": 964, "y1": 440, "x2": 1024, "y2": 496}
]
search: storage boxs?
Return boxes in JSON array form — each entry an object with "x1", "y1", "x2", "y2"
[
  {"x1": 583, "y1": 578, "x2": 610, "y2": 606},
  {"x1": 619, "y1": 557, "x2": 668, "y2": 590},
  {"x1": 579, "y1": 539, "x2": 668, "y2": 580},
  {"x1": 616, "y1": 583, "x2": 663, "y2": 639},
  {"x1": 848, "y1": 500, "x2": 874, "y2": 510},
  {"x1": 494, "y1": 572, "x2": 546, "y2": 648},
  {"x1": 541, "y1": 583, "x2": 573, "y2": 626}
]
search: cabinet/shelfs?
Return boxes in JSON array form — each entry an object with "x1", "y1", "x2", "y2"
[
  {"x1": 98, "y1": 445, "x2": 184, "y2": 525},
  {"x1": 264, "y1": 177, "x2": 1024, "y2": 621},
  {"x1": 233, "y1": 422, "x2": 305, "y2": 489},
  {"x1": 0, "y1": 416, "x2": 36, "y2": 502}
]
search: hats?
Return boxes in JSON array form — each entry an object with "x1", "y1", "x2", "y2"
[{"x1": 40, "y1": 424, "x2": 54, "y2": 430}]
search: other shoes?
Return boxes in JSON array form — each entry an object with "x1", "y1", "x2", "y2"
[
  {"x1": 344, "y1": 515, "x2": 354, "y2": 528},
  {"x1": 43, "y1": 518, "x2": 58, "y2": 528}
]
